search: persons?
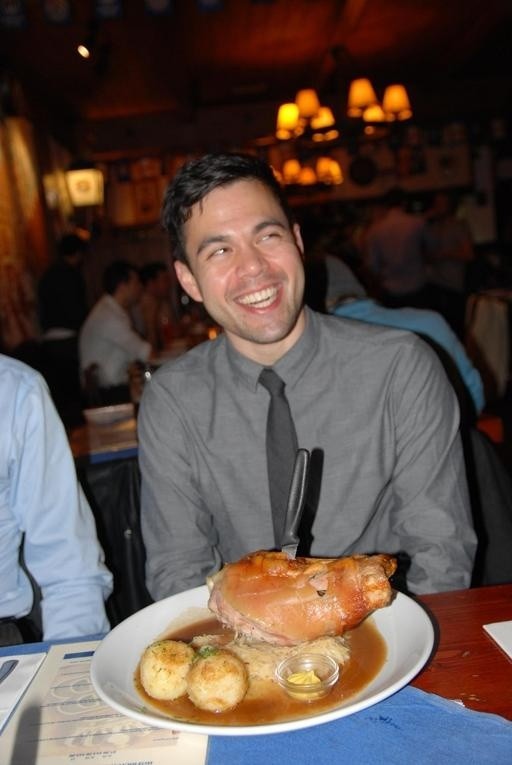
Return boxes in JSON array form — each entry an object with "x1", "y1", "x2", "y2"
[
  {"x1": 0, "y1": 350, "x2": 119, "y2": 647},
  {"x1": 39, "y1": 234, "x2": 95, "y2": 365},
  {"x1": 78, "y1": 259, "x2": 152, "y2": 419},
  {"x1": 293, "y1": 186, "x2": 472, "y2": 312},
  {"x1": 132, "y1": 264, "x2": 212, "y2": 343},
  {"x1": 134, "y1": 151, "x2": 485, "y2": 602}
]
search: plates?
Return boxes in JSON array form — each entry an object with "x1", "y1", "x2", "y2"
[
  {"x1": 82, "y1": 403, "x2": 135, "y2": 427},
  {"x1": 90, "y1": 583, "x2": 435, "y2": 736}
]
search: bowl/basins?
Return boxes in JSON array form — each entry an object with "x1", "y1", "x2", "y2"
[{"x1": 274, "y1": 651, "x2": 339, "y2": 702}]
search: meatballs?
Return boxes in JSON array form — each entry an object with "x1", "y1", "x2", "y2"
[
  {"x1": 187, "y1": 651, "x2": 248, "y2": 712},
  {"x1": 139, "y1": 639, "x2": 198, "y2": 700}
]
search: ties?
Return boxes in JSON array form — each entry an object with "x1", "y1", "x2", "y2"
[{"x1": 258, "y1": 369, "x2": 297, "y2": 548}]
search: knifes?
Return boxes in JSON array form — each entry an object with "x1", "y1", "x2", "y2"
[{"x1": 280, "y1": 448, "x2": 311, "y2": 560}]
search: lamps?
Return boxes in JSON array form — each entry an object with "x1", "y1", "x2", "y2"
[{"x1": 266, "y1": 49, "x2": 413, "y2": 145}]
